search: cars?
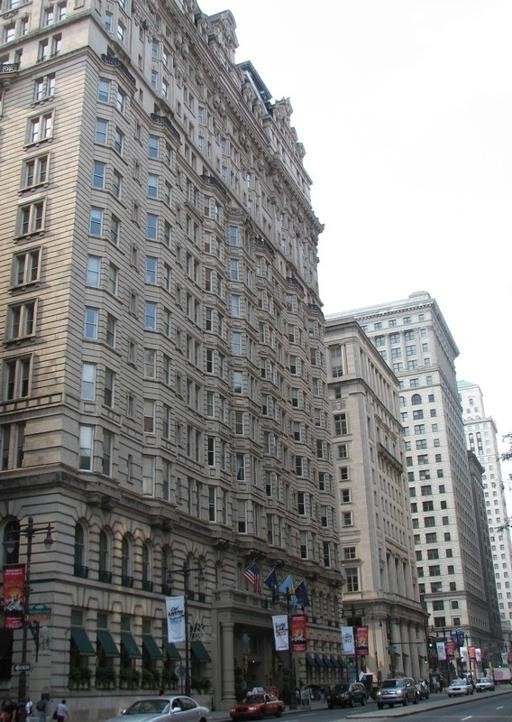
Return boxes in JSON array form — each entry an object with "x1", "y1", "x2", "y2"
[
  {"x1": 108, "y1": 695, "x2": 212, "y2": 721},
  {"x1": 231, "y1": 685, "x2": 286, "y2": 719},
  {"x1": 473, "y1": 676, "x2": 495, "y2": 693},
  {"x1": 446, "y1": 678, "x2": 474, "y2": 697},
  {"x1": 414, "y1": 683, "x2": 429, "y2": 700},
  {"x1": 370, "y1": 686, "x2": 378, "y2": 700}
]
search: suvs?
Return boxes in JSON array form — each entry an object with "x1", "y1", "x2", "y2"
[
  {"x1": 327, "y1": 682, "x2": 369, "y2": 708},
  {"x1": 375, "y1": 676, "x2": 417, "y2": 710}
]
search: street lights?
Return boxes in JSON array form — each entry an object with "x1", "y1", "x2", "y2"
[
  {"x1": 435, "y1": 626, "x2": 455, "y2": 681},
  {"x1": 378, "y1": 613, "x2": 401, "y2": 678},
  {"x1": 339, "y1": 603, "x2": 367, "y2": 680},
  {"x1": 498, "y1": 642, "x2": 512, "y2": 653},
  {"x1": 3, "y1": 516, "x2": 54, "y2": 721},
  {"x1": 272, "y1": 585, "x2": 305, "y2": 710},
  {"x1": 166, "y1": 560, "x2": 207, "y2": 696},
  {"x1": 458, "y1": 633, "x2": 481, "y2": 679}
]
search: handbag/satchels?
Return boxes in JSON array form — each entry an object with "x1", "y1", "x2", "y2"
[{"x1": 53, "y1": 711, "x2": 58, "y2": 720}]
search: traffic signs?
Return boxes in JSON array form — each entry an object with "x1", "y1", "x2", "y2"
[{"x1": 14, "y1": 662, "x2": 31, "y2": 672}]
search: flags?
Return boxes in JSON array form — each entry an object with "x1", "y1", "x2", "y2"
[
  {"x1": 263, "y1": 569, "x2": 278, "y2": 604},
  {"x1": 278, "y1": 575, "x2": 297, "y2": 606},
  {"x1": 295, "y1": 581, "x2": 311, "y2": 608},
  {"x1": 242, "y1": 560, "x2": 261, "y2": 594}
]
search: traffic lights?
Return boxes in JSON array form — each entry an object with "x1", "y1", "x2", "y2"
[{"x1": 427, "y1": 640, "x2": 432, "y2": 648}]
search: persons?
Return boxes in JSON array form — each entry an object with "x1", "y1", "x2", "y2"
[
  {"x1": 295, "y1": 685, "x2": 331, "y2": 706},
  {"x1": 0, "y1": 695, "x2": 70, "y2": 722}
]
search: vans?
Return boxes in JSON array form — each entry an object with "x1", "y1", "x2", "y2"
[{"x1": 429, "y1": 671, "x2": 445, "y2": 694}]
